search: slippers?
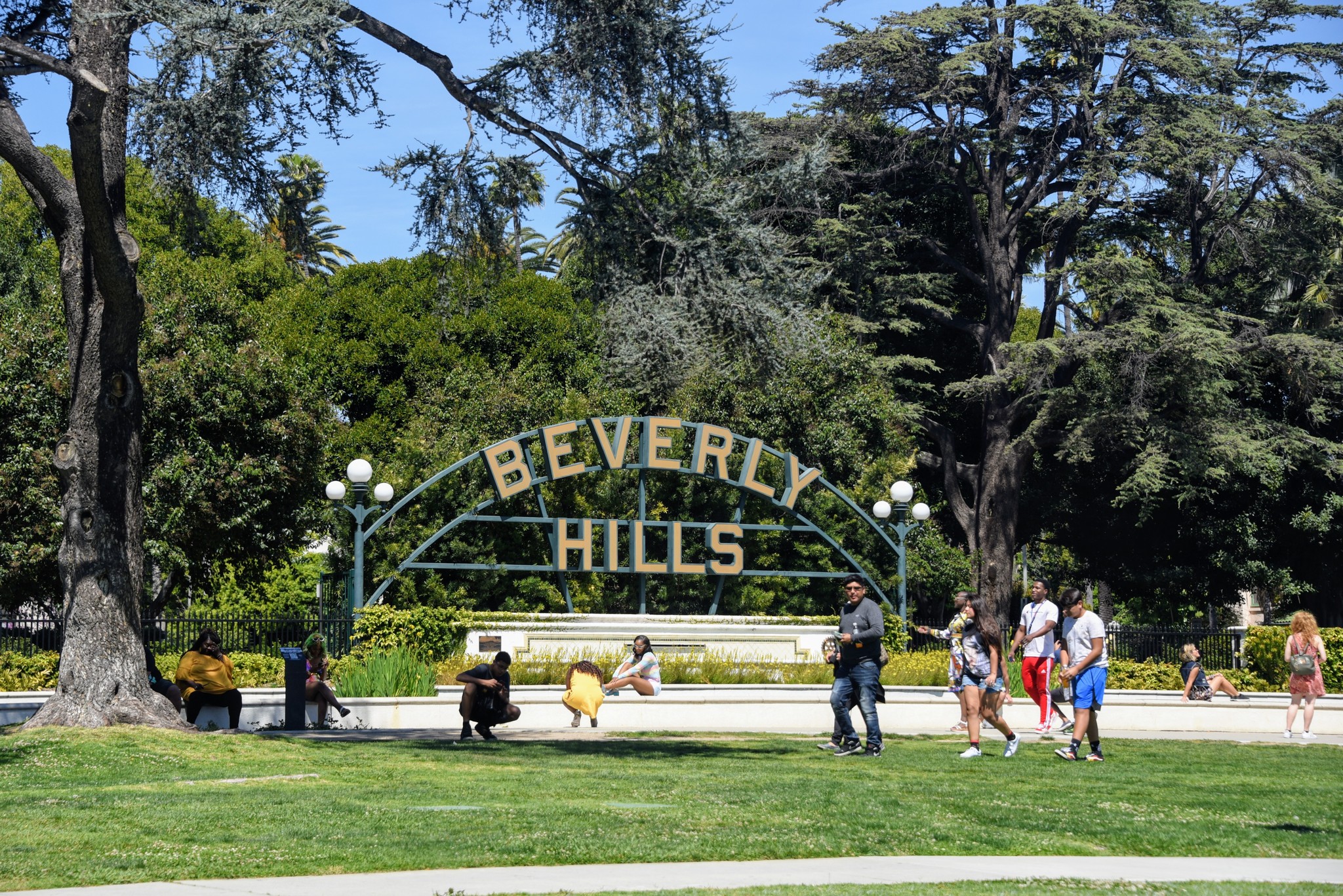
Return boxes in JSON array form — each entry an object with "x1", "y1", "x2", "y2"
[{"x1": 340, "y1": 707, "x2": 351, "y2": 717}]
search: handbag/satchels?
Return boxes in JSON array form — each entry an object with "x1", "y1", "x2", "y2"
[{"x1": 870, "y1": 642, "x2": 888, "y2": 669}]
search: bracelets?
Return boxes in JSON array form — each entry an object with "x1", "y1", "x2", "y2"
[
  {"x1": 1063, "y1": 664, "x2": 1067, "y2": 667},
  {"x1": 1209, "y1": 674, "x2": 1214, "y2": 680},
  {"x1": 217, "y1": 652, "x2": 222, "y2": 659}
]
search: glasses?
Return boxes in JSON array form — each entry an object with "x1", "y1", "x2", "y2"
[
  {"x1": 633, "y1": 642, "x2": 646, "y2": 649},
  {"x1": 496, "y1": 661, "x2": 509, "y2": 672},
  {"x1": 312, "y1": 638, "x2": 325, "y2": 641},
  {"x1": 845, "y1": 587, "x2": 863, "y2": 592},
  {"x1": 955, "y1": 597, "x2": 965, "y2": 600}
]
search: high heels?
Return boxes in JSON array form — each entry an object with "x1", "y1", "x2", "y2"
[
  {"x1": 571, "y1": 710, "x2": 582, "y2": 727},
  {"x1": 600, "y1": 684, "x2": 619, "y2": 696},
  {"x1": 590, "y1": 718, "x2": 598, "y2": 727},
  {"x1": 950, "y1": 720, "x2": 968, "y2": 732}
]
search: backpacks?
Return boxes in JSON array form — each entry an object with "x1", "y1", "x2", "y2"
[{"x1": 1290, "y1": 635, "x2": 1316, "y2": 676}]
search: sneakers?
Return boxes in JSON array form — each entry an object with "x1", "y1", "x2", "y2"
[
  {"x1": 1054, "y1": 747, "x2": 1077, "y2": 761},
  {"x1": 460, "y1": 727, "x2": 474, "y2": 740},
  {"x1": 474, "y1": 724, "x2": 498, "y2": 741},
  {"x1": 1003, "y1": 733, "x2": 1020, "y2": 757},
  {"x1": 1230, "y1": 693, "x2": 1250, "y2": 701},
  {"x1": 1079, "y1": 753, "x2": 1103, "y2": 761},
  {"x1": 860, "y1": 743, "x2": 881, "y2": 757},
  {"x1": 834, "y1": 736, "x2": 862, "y2": 756},
  {"x1": 1048, "y1": 710, "x2": 1059, "y2": 731},
  {"x1": 1284, "y1": 730, "x2": 1318, "y2": 739},
  {"x1": 1034, "y1": 724, "x2": 1048, "y2": 734},
  {"x1": 959, "y1": 747, "x2": 982, "y2": 758},
  {"x1": 1063, "y1": 722, "x2": 1075, "y2": 735}
]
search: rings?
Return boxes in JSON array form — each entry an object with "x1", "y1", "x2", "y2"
[
  {"x1": 991, "y1": 681, "x2": 992, "y2": 683},
  {"x1": 196, "y1": 686, "x2": 198, "y2": 688},
  {"x1": 213, "y1": 648, "x2": 214, "y2": 652},
  {"x1": 195, "y1": 687, "x2": 196, "y2": 689}
]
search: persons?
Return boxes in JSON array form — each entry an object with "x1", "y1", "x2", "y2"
[
  {"x1": 917, "y1": 591, "x2": 1020, "y2": 758},
  {"x1": 302, "y1": 633, "x2": 350, "y2": 730},
  {"x1": 562, "y1": 661, "x2": 605, "y2": 727},
  {"x1": 456, "y1": 652, "x2": 521, "y2": 741},
  {"x1": 1285, "y1": 611, "x2": 1327, "y2": 738},
  {"x1": 1179, "y1": 643, "x2": 1250, "y2": 703},
  {"x1": 143, "y1": 647, "x2": 182, "y2": 714},
  {"x1": 1050, "y1": 589, "x2": 1110, "y2": 763},
  {"x1": 1007, "y1": 578, "x2": 1058, "y2": 734},
  {"x1": 600, "y1": 635, "x2": 661, "y2": 696},
  {"x1": 816, "y1": 574, "x2": 888, "y2": 757},
  {"x1": 176, "y1": 628, "x2": 242, "y2": 730}
]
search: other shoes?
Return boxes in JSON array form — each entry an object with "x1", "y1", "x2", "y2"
[
  {"x1": 982, "y1": 721, "x2": 994, "y2": 728},
  {"x1": 816, "y1": 742, "x2": 839, "y2": 750},
  {"x1": 880, "y1": 741, "x2": 885, "y2": 751},
  {"x1": 1057, "y1": 720, "x2": 1071, "y2": 731}
]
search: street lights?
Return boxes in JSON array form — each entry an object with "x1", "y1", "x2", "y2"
[
  {"x1": 873, "y1": 480, "x2": 931, "y2": 653},
  {"x1": 324, "y1": 458, "x2": 394, "y2": 623}
]
